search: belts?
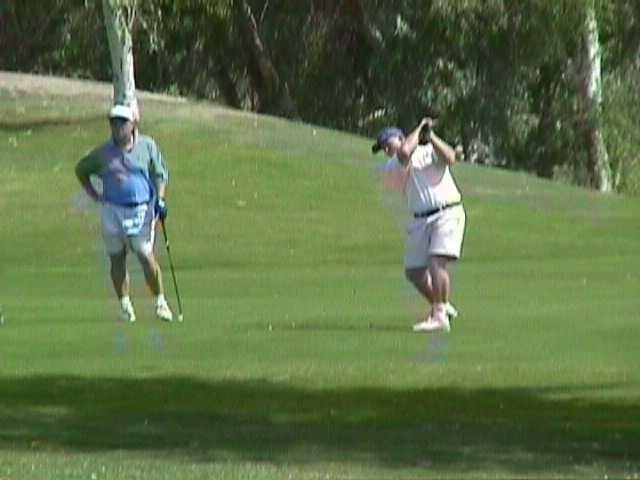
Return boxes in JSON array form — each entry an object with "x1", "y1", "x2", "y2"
[{"x1": 413, "y1": 200, "x2": 461, "y2": 217}]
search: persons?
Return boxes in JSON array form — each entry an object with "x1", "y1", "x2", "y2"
[
  {"x1": 372, "y1": 114, "x2": 468, "y2": 334},
  {"x1": 75, "y1": 104, "x2": 185, "y2": 323}
]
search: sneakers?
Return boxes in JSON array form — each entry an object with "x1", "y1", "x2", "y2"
[
  {"x1": 120, "y1": 301, "x2": 136, "y2": 322},
  {"x1": 412, "y1": 302, "x2": 458, "y2": 334},
  {"x1": 155, "y1": 300, "x2": 173, "y2": 321}
]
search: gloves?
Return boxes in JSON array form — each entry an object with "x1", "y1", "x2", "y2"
[{"x1": 154, "y1": 196, "x2": 168, "y2": 221}]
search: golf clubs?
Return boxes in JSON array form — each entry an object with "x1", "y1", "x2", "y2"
[{"x1": 162, "y1": 218, "x2": 183, "y2": 322}]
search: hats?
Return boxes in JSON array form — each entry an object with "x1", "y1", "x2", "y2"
[
  {"x1": 372, "y1": 127, "x2": 402, "y2": 154},
  {"x1": 107, "y1": 104, "x2": 135, "y2": 122}
]
